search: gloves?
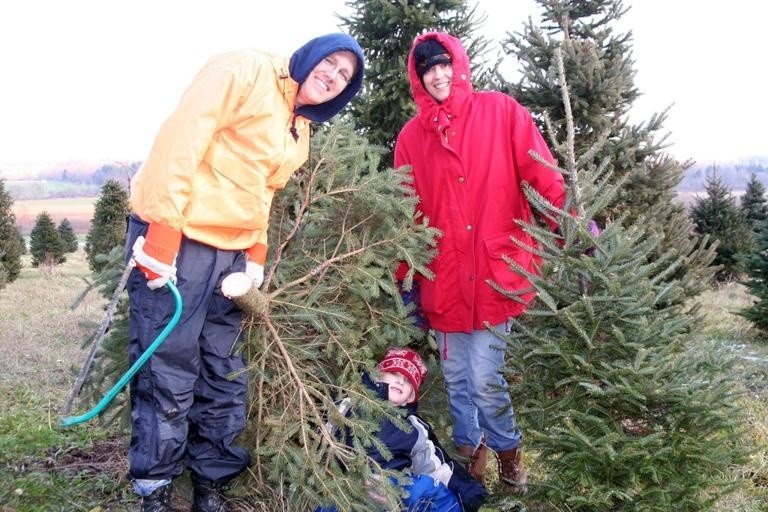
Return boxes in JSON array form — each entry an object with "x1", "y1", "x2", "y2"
[
  {"x1": 133, "y1": 223, "x2": 182, "y2": 290},
  {"x1": 246, "y1": 242, "x2": 269, "y2": 288}
]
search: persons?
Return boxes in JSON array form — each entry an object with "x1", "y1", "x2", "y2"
[
  {"x1": 390, "y1": 32, "x2": 603, "y2": 497},
  {"x1": 310, "y1": 349, "x2": 490, "y2": 512},
  {"x1": 124, "y1": 33, "x2": 366, "y2": 512}
]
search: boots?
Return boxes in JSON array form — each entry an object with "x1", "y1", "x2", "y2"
[
  {"x1": 138, "y1": 480, "x2": 182, "y2": 512},
  {"x1": 483, "y1": 439, "x2": 528, "y2": 496},
  {"x1": 189, "y1": 474, "x2": 231, "y2": 512},
  {"x1": 451, "y1": 434, "x2": 488, "y2": 489}
]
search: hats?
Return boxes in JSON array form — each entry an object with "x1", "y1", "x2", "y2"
[
  {"x1": 414, "y1": 39, "x2": 453, "y2": 81},
  {"x1": 375, "y1": 344, "x2": 428, "y2": 403}
]
